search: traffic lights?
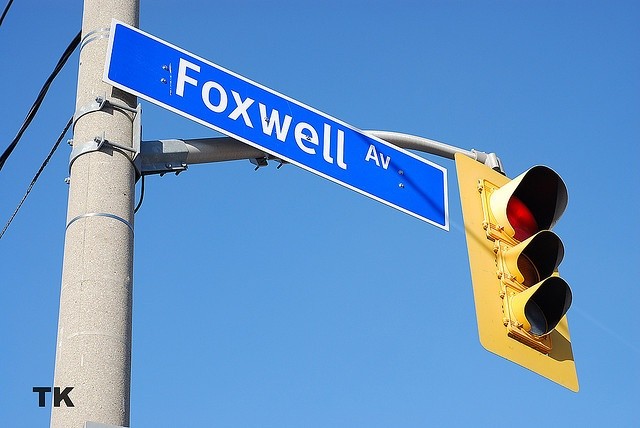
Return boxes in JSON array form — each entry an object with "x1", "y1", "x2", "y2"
[{"x1": 481, "y1": 163, "x2": 576, "y2": 360}]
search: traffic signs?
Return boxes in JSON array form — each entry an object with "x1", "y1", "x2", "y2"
[{"x1": 103, "y1": 18, "x2": 450, "y2": 231}]
